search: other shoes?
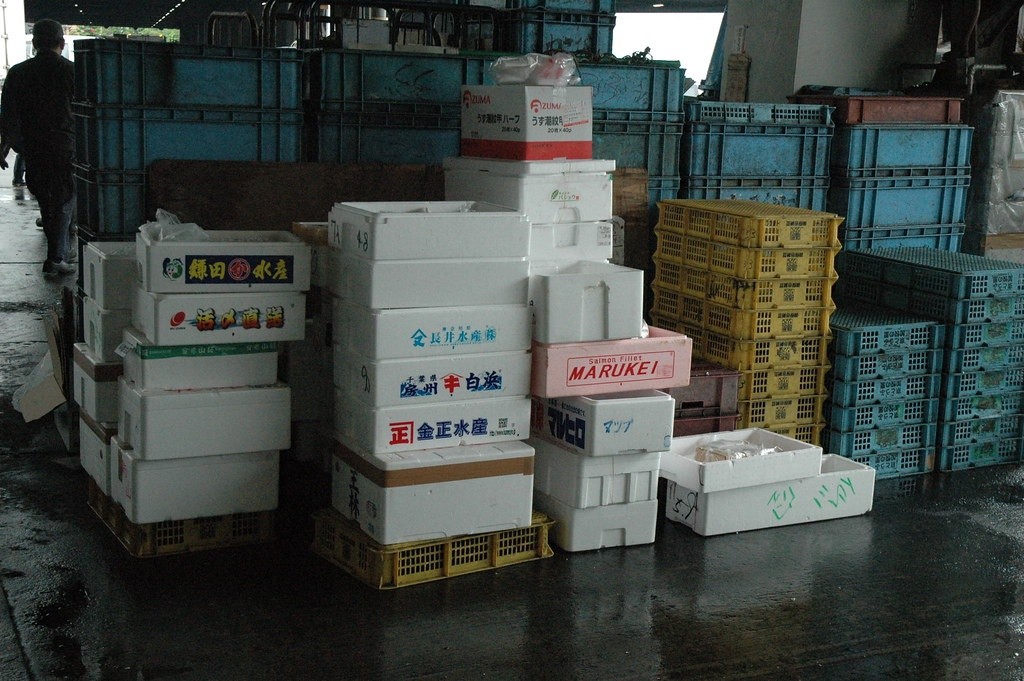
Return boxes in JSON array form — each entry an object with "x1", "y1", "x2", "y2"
[
  {"x1": 43, "y1": 259, "x2": 75, "y2": 273},
  {"x1": 36, "y1": 217, "x2": 43, "y2": 226},
  {"x1": 12, "y1": 179, "x2": 26, "y2": 186}
]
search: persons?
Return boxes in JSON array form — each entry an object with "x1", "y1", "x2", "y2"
[{"x1": 0, "y1": 18, "x2": 79, "y2": 277}]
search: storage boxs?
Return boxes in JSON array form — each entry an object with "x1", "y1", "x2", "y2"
[{"x1": 40, "y1": 0, "x2": 1024, "y2": 592}]
